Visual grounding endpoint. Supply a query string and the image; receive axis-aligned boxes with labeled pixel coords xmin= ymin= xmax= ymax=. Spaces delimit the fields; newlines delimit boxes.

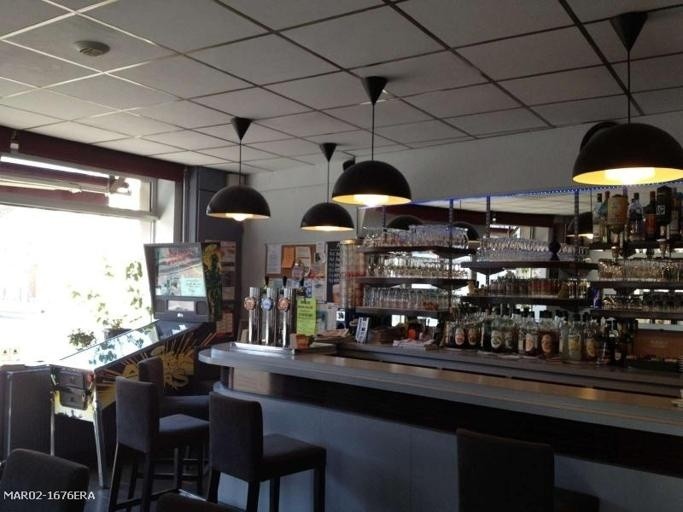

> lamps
xmin=300 ymin=143 xmax=354 ymax=231
xmin=207 ymin=117 xmax=270 ymax=221
xmin=331 ymin=76 xmax=413 ymax=206
xmin=573 ymin=11 xmax=683 ymax=186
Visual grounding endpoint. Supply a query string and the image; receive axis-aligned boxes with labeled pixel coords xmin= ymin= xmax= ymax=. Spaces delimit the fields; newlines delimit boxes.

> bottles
xmin=593 ymin=186 xmax=681 ymax=244
xmin=448 ymin=260 xmax=682 ymax=365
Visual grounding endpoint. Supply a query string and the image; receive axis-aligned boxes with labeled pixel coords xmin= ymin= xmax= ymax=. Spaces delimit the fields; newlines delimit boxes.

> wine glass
xmin=364 ymin=227 xmax=469 ymax=309
xmin=477 ymin=236 xmax=575 ymax=259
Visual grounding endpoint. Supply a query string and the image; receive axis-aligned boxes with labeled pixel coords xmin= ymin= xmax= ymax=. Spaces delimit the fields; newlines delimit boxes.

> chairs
xmin=0 ymin=448 xmax=90 ymax=511
xmin=455 ymin=429 xmax=599 ymax=512
xmin=123 ymin=357 xmax=210 ymax=511
xmin=0 ymin=365 xmax=49 ymax=454
xmin=208 ymin=391 xmax=325 ymax=511
xmin=107 ymin=378 xmax=209 ymax=511
